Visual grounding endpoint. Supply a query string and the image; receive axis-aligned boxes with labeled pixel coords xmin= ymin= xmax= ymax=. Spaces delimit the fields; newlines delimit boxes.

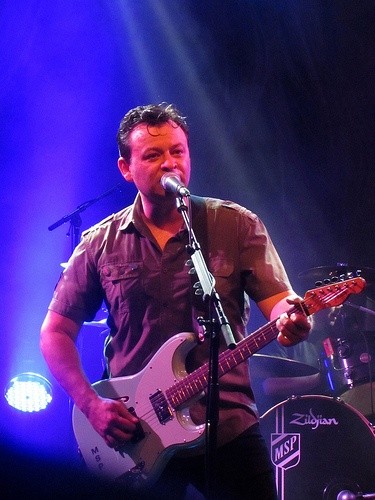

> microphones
xmin=160 ymin=172 xmax=191 ymax=197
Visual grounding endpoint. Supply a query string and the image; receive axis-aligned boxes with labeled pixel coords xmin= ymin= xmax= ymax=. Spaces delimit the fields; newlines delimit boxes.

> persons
xmin=40 ymin=102 xmax=313 ymax=500
xmin=261 ymin=304 xmax=349 ymax=398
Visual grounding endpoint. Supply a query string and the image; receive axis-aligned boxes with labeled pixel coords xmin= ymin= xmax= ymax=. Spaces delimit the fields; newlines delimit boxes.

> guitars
xmin=73 ymin=270 xmax=366 ymax=496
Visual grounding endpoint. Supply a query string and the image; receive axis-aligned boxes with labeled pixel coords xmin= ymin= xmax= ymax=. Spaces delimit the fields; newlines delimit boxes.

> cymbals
xmin=247 ymin=353 xmax=319 ymax=378
xmin=299 ymin=263 xmax=374 ymax=283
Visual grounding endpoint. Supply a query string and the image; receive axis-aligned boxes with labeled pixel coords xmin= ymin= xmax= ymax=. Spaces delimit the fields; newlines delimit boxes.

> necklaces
xmin=179 ymin=221 xmax=185 ymax=232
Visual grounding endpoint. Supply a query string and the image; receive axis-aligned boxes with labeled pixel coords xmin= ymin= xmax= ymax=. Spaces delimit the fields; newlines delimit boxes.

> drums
xmin=256 ymin=395 xmax=375 ymax=500
xmin=331 ymin=378 xmax=374 ymax=428
